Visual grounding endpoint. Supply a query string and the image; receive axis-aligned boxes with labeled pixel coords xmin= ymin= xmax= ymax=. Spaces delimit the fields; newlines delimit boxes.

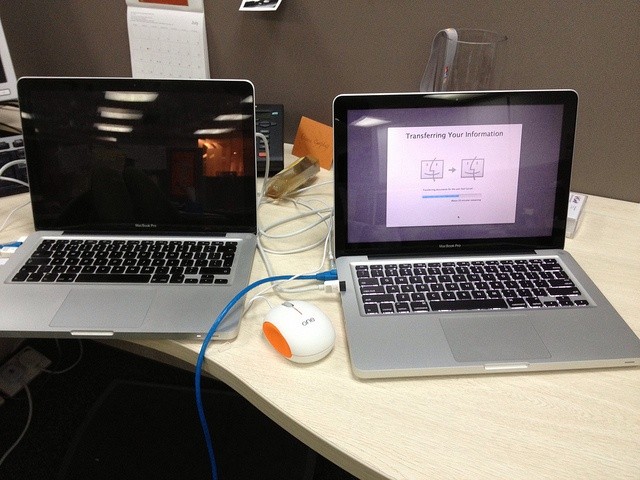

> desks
xmin=0 ymin=143 xmax=640 ymax=479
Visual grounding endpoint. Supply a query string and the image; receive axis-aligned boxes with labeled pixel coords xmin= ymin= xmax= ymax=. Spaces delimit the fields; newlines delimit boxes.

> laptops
xmin=1 ymin=75 xmax=258 ymax=341
xmin=331 ymin=88 xmax=640 ymax=380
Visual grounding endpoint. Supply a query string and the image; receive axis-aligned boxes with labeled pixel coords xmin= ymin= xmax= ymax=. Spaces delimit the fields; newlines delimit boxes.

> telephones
xmin=254 ymin=103 xmax=284 ymax=177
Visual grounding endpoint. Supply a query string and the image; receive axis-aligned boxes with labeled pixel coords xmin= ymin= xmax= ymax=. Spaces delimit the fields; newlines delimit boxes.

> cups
xmin=419 ymin=29 xmax=509 ymax=92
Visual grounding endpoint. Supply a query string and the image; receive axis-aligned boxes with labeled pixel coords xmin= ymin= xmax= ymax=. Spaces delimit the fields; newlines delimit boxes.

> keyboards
xmin=0 ymin=148 xmax=29 ymax=198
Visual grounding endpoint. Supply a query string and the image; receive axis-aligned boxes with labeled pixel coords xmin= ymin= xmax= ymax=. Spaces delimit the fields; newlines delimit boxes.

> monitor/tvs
xmin=0 ymin=18 xmax=18 ymax=102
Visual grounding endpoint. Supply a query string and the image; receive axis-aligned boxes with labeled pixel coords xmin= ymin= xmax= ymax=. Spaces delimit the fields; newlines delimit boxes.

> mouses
xmin=263 ymin=300 xmax=335 ymax=364
